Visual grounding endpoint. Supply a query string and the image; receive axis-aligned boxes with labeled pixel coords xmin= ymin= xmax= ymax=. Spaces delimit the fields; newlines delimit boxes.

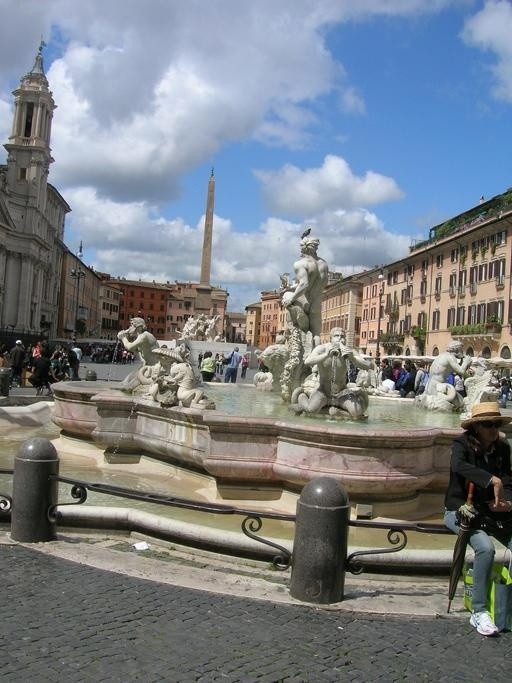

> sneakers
xmin=469 ymin=610 xmax=499 ymax=636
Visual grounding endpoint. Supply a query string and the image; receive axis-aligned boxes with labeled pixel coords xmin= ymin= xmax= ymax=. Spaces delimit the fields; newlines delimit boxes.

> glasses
xmin=478 ymin=419 xmax=503 ymax=429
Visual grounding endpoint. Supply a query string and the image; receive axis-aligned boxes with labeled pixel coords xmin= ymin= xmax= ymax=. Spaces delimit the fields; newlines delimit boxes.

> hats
xmin=461 ymin=402 xmax=511 ymax=428
xmin=16 ymin=340 xmax=22 ymax=345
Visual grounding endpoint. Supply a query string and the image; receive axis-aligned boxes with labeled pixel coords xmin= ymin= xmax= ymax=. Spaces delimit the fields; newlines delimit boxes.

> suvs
xmin=47 ymin=337 xmax=76 ymax=356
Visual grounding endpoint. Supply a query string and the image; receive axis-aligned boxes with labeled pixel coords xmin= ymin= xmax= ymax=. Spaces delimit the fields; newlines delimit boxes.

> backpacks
xmin=222 ymin=351 xmax=234 ymax=364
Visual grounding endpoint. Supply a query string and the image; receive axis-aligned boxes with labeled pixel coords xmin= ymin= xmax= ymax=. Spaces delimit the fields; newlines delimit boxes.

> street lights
xmin=266 ymin=317 xmax=271 ymax=346
xmin=375 ymin=272 xmax=385 ymax=366
xmin=70 ymin=236 xmax=87 ymax=349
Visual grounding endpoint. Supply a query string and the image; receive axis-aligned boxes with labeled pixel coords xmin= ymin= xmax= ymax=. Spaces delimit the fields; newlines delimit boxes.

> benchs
xmin=415 ymin=519 xmax=458 ymax=536
xmin=85 ymin=479 xmax=237 ymax=520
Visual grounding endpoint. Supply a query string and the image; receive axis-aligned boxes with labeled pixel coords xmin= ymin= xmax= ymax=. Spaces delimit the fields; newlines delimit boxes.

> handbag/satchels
xmin=455 ymin=499 xmax=511 ymax=538
xmin=463 ymin=548 xmax=512 ymax=633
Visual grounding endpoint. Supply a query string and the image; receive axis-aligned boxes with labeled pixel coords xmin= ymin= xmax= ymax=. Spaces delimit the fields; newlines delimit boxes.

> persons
xmin=197 ymin=347 xmax=249 ymax=383
xmin=443 ymin=402 xmax=512 ymax=637
xmin=170 ymin=343 xmax=205 ymax=409
xmin=281 ymin=237 xmax=329 ymax=334
xmin=381 ymin=357 xmax=512 ymax=402
xmin=423 ymin=340 xmax=473 ymax=407
xmin=0 ymin=339 xmax=136 ymax=395
xmin=117 ymin=317 xmax=162 ymax=387
xmin=298 ymin=327 xmax=373 ymax=417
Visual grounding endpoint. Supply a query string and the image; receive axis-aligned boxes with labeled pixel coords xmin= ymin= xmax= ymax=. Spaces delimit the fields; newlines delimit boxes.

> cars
xmin=90 ymin=350 xmax=107 ymax=361
